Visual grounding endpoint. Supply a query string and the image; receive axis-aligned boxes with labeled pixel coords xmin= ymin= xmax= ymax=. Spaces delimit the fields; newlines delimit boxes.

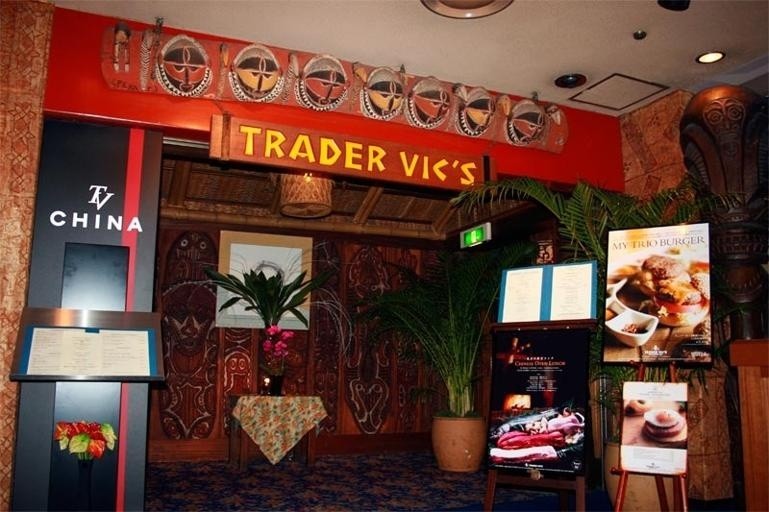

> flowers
xmin=185 ymin=240 xmax=354 ymax=366
xmin=53 ymin=420 xmax=118 ymax=460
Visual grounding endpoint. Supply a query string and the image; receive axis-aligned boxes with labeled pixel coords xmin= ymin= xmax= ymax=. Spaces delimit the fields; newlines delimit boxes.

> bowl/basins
xmin=605 ymin=308 xmax=658 ymax=348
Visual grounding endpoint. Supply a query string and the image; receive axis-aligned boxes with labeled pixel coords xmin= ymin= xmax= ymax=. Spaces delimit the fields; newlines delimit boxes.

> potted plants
xmin=448 ymin=174 xmax=747 ymax=512
xmin=354 ymin=239 xmax=540 ymax=473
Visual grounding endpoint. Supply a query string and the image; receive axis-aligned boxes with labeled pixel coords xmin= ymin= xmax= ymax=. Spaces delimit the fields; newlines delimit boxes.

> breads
xmin=603 ymin=251 xmax=710 ymax=326
xmin=624 ymin=398 xmax=688 ymax=443
xmin=489 ymin=405 xmax=588 ymax=468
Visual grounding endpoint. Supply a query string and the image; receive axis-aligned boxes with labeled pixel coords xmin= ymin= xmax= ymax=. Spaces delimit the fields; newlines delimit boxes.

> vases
xmin=259 ymin=353 xmax=289 ymax=396
xmin=77 ymin=460 xmax=93 ymax=512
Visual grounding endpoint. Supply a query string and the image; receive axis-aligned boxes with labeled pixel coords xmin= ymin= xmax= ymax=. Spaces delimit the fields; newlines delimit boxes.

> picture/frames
xmin=215 ymin=230 xmax=313 ymax=330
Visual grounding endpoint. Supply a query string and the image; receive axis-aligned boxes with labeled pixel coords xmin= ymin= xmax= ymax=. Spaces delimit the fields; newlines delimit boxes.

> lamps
xmin=279 ymin=167 xmax=335 ymax=219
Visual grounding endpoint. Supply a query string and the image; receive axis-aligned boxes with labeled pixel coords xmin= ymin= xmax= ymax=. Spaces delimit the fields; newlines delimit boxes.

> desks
xmin=228 ymin=393 xmax=328 ymax=473
